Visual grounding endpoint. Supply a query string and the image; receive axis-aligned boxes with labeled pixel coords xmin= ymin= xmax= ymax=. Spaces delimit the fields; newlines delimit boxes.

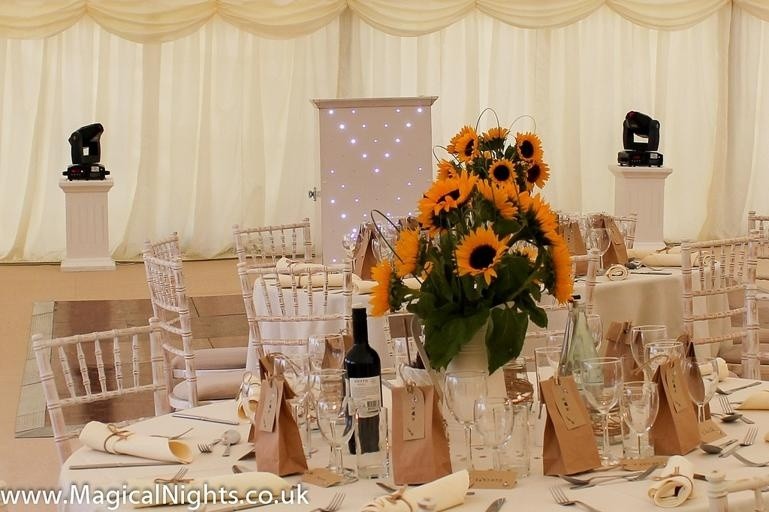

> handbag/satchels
xmin=391 ymin=387 xmax=452 ymax=485
xmin=648 ymin=357 xmax=701 ymax=456
xmin=590 ymin=214 xmax=629 ymax=268
xmin=321 ymin=329 xmax=354 ymax=415
xmin=559 ymin=221 xmax=588 ymax=277
xmin=539 ymin=375 xmax=601 ymax=476
xmin=247 ymin=353 xmax=299 ymax=443
xmin=352 ymin=224 xmax=381 ymax=281
xmin=255 ymin=378 xmax=308 ymax=476
xmin=605 ymin=321 xmax=643 ymax=387
xmin=671 ymin=335 xmax=711 ymax=423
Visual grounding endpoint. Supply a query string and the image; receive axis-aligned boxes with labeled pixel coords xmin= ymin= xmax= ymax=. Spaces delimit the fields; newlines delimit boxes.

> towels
xmin=648 ymin=455 xmax=695 ymax=508
xmin=360 ymin=470 xmax=469 ymax=512
xmin=132 ymin=471 xmax=290 ymax=507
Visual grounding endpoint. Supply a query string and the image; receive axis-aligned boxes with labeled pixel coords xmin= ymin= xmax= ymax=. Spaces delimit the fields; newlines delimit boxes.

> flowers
xmin=367 ymin=108 xmax=576 ymax=377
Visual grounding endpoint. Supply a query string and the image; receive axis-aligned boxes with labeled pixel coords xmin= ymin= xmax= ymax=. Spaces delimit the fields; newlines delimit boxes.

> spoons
xmin=231 ymin=464 xmax=253 ymax=475
xmin=699 ymin=440 xmax=737 ymax=454
xmin=221 ymin=429 xmax=241 ymax=456
xmin=376 ymin=481 xmax=476 ymax=497
xmin=711 ymin=412 xmax=743 ymax=423
xmin=556 ymin=473 xmax=644 ymax=485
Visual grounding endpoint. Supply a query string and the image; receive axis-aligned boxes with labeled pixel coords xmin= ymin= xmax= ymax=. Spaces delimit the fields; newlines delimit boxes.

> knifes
xmin=210 ymin=498 xmax=290 ymax=512
xmin=172 ymin=411 xmax=239 ymax=427
xmin=485 ymin=497 xmax=507 ymax=512
xmin=68 ymin=461 xmax=183 ymax=470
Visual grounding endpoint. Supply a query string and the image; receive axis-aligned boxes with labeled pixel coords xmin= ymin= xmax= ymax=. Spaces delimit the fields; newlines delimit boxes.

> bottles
xmin=343 ymin=307 xmax=383 ymax=455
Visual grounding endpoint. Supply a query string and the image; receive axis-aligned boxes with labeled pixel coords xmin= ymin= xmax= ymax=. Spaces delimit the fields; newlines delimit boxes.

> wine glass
xmin=580 ymin=356 xmax=624 ymax=457
xmin=586 ymin=313 xmax=603 ymax=353
xmin=544 ymin=334 xmax=563 ymax=369
xmin=473 ymin=397 xmax=515 ymax=471
xmin=618 ymin=380 xmax=660 ymax=456
xmin=443 ymin=369 xmax=489 ymax=467
xmin=341 ymin=216 xmax=399 ymax=269
xmin=557 ymin=210 xmax=612 ymax=273
xmin=629 ymin=324 xmax=667 ymax=371
xmin=308 ymin=368 xmax=351 ymax=475
xmin=642 ymin=341 xmax=684 ymax=378
xmin=683 ymin=356 xmax=719 ymax=423
xmin=315 ymin=393 xmax=359 ymax=487
xmin=307 ymin=333 xmax=346 ymax=370
xmin=273 ymin=353 xmax=315 ymax=423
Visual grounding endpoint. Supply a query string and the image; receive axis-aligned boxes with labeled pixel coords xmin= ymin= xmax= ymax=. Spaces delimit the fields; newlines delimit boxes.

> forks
xmin=170 ymin=466 xmax=189 ymax=481
xmin=717 ymin=381 xmax=762 ymax=395
xmin=568 ymin=463 xmax=659 ymax=490
xmin=718 ymin=427 xmax=759 ymax=458
xmin=149 ymin=427 xmax=194 ymax=440
xmin=310 ymin=491 xmax=346 ymax=512
xmin=549 ymin=485 xmax=601 ymax=511
xmin=718 ymin=395 xmax=754 ymax=424
xmin=197 ymin=437 xmax=221 ymax=454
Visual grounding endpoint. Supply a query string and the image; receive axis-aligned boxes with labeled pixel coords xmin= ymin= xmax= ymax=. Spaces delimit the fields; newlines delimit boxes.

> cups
xmin=533 ymin=346 xmax=561 ymax=400
xmin=355 ymin=406 xmax=389 ymax=480
xmin=492 ymin=404 xmax=531 ymax=480
